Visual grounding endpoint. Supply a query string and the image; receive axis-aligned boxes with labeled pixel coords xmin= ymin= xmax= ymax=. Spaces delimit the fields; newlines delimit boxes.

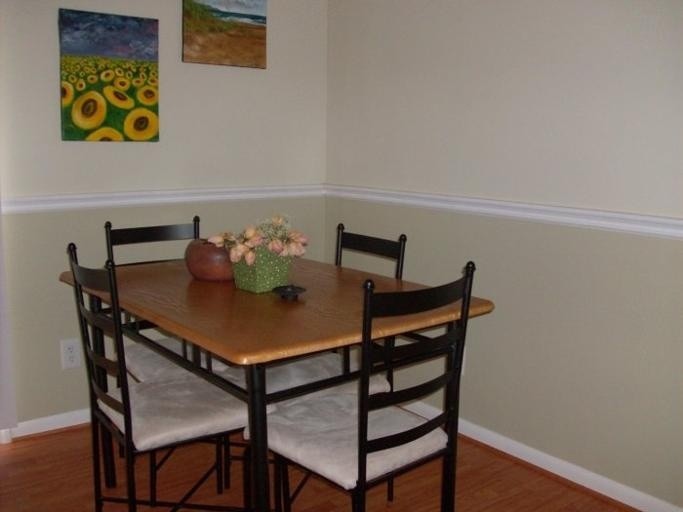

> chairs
xmin=243 ymin=257 xmax=475 ymax=512
xmin=68 ymin=242 xmax=283 ymax=512
xmin=259 ymin=222 xmax=407 ymax=501
xmin=104 ymin=218 xmax=240 ymax=490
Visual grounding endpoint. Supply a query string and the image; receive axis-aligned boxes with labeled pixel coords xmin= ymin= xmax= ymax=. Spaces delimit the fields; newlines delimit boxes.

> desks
xmin=61 ymin=253 xmax=494 ymax=512
xmin=58 ymin=257 xmax=495 ymax=512
